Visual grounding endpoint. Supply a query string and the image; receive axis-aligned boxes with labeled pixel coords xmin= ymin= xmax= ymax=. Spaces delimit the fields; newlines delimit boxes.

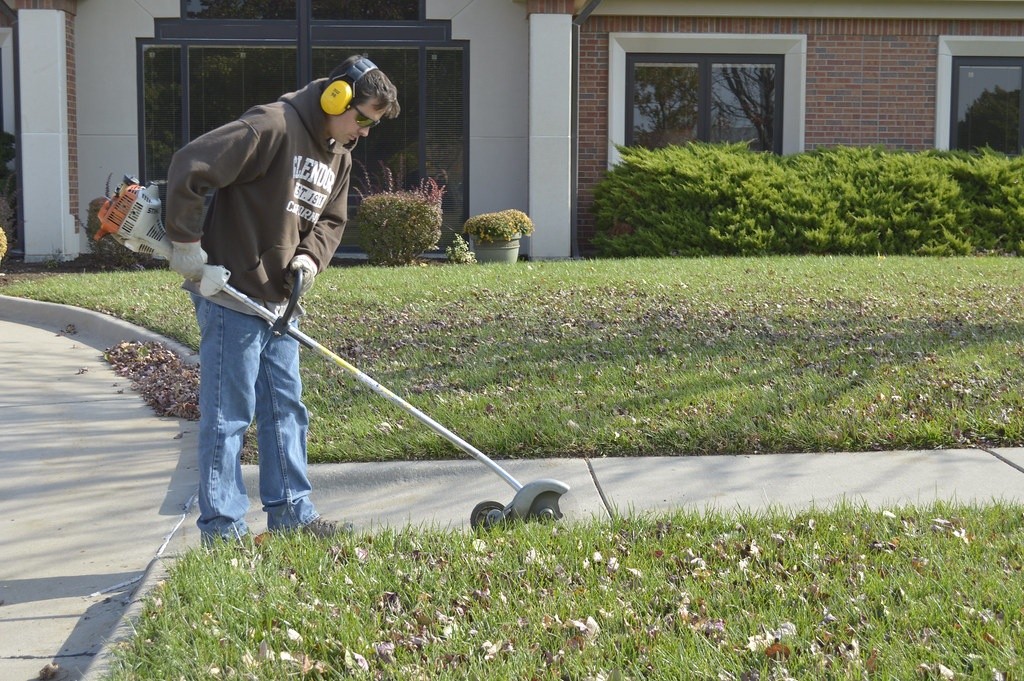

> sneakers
xmin=302 ymin=518 xmax=356 ymax=538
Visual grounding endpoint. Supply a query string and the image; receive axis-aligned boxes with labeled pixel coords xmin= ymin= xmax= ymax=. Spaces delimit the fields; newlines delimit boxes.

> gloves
xmin=169 ymin=239 xmax=208 ymax=279
xmin=283 ymin=253 xmax=319 ymax=296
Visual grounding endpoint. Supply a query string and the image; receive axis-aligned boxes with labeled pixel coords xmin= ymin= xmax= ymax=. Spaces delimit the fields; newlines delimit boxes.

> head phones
xmin=318 ymin=58 xmax=378 ymax=116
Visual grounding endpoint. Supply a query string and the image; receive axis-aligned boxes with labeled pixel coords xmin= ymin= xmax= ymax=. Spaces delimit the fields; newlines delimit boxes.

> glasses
xmin=352 ymin=104 xmax=380 ymax=129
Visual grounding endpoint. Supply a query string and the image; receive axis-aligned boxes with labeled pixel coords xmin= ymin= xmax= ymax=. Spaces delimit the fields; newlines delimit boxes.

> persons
xmin=164 ymin=55 xmax=401 ymax=551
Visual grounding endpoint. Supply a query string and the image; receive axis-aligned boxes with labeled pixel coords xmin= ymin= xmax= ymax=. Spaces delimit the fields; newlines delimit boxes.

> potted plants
xmin=461 ymin=209 xmax=535 ymax=264
